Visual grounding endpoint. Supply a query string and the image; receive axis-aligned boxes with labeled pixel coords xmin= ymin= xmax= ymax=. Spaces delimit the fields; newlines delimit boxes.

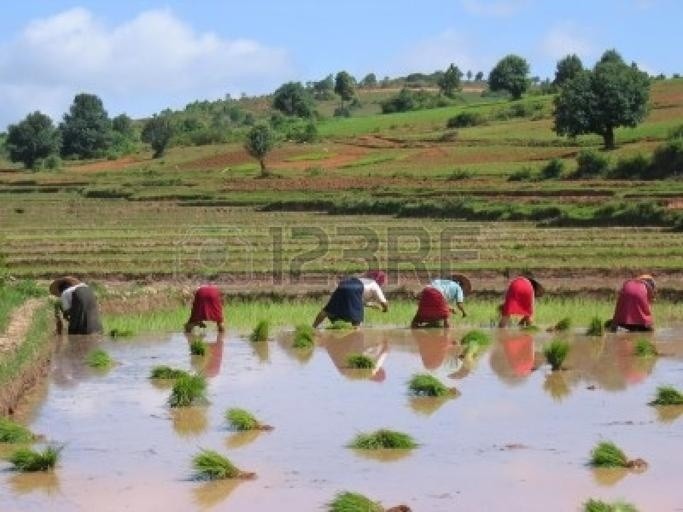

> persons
xmin=492 ymin=273 xmax=546 ymax=330
xmin=605 ymin=272 xmax=658 ymax=335
xmin=310 ymin=266 xmax=389 ymax=330
xmin=488 ymin=329 xmax=546 ymax=381
xmin=181 ymin=282 xmax=226 ymax=336
xmin=47 ymin=275 xmax=106 ymax=336
xmin=408 ymin=274 xmax=473 ymax=329
xmin=316 ymin=328 xmax=390 ymax=384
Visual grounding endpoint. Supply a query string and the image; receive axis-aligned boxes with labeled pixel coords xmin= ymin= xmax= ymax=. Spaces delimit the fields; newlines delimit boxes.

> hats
xmin=49 ymin=276 xmax=80 ymax=298
xmin=444 ymin=271 xmax=472 ymax=298
